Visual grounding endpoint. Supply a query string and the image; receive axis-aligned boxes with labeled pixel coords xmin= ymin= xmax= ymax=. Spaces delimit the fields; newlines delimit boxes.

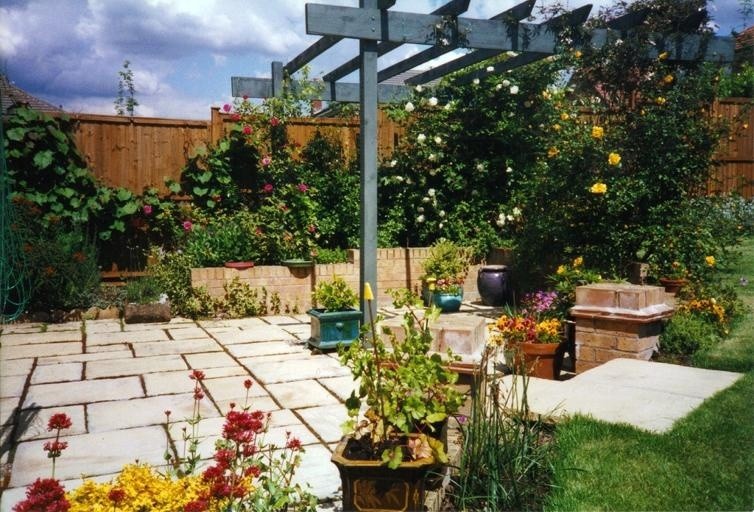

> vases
xmin=515 ymin=341 xmax=563 ymax=381
xmin=432 ymin=291 xmax=463 ymax=312
xmin=477 ymin=263 xmax=510 ymax=307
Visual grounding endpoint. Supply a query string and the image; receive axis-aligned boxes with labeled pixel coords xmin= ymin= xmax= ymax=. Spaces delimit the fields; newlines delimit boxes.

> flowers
xmin=433 ymin=276 xmax=466 ymax=297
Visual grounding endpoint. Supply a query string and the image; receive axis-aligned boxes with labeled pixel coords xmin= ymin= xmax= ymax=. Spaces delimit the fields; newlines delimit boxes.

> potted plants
xmin=330 ymin=293 xmax=473 ymax=512
xmin=306 ymin=272 xmax=365 ymax=355
